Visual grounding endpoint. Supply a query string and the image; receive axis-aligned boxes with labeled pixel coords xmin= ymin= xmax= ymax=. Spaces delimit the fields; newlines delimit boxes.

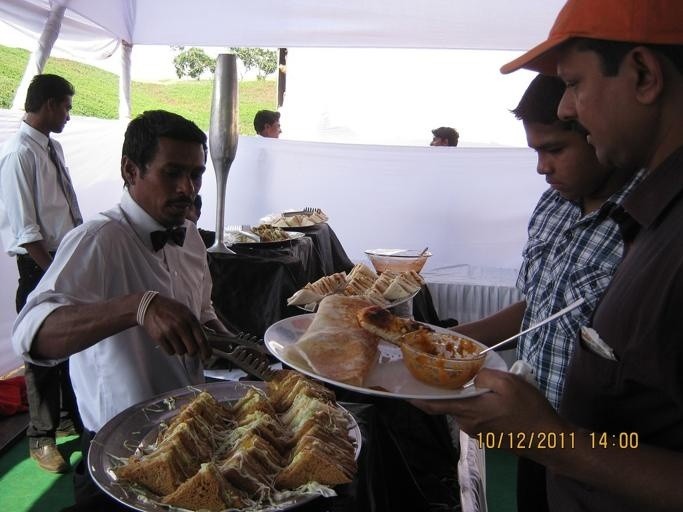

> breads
xmin=286 ymin=293 xmax=386 ymax=387
xmin=356 ymin=304 xmax=435 ymax=347
xmin=248 ymin=223 xmax=291 ymax=242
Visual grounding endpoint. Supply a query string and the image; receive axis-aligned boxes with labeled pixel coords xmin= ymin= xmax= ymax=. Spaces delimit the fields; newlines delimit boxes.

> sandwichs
xmin=261 ymin=206 xmax=328 ymax=228
xmin=286 ymin=262 xmax=427 ymax=307
xmin=113 ymin=368 xmax=359 ymax=512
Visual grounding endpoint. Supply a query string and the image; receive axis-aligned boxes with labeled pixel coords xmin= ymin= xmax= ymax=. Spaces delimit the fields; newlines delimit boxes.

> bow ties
xmin=149 ymin=227 xmax=186 ymax=253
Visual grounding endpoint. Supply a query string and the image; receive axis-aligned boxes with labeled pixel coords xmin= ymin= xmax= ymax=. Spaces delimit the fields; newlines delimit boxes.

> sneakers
xmin=28 ymin=443 xmax=68 ymax=473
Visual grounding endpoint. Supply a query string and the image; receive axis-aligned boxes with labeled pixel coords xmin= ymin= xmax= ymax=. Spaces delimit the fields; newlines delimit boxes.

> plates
xmin=225 ymin=231 xmax=306 ymax=244
xmin=87 ymin=378 xmax=363 ymax=511
xmin=295 ymin=280 xmax=419 ymax=312
xmin=264 ymin=314 xmax=509 ymax=401
xmin=261 ymin=211 xmax=329 ymax=227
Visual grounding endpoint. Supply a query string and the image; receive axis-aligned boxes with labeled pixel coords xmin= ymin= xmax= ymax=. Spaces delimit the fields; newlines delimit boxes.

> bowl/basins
xmin=402 ymin=331 xmax=489 ymax=388
xmin=364 ymin=248 xmax=432 ymax=274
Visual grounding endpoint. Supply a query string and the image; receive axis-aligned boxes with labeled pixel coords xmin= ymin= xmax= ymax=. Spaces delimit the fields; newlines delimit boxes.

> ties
xmin=47 ymin=139 xmax=83 ymax=227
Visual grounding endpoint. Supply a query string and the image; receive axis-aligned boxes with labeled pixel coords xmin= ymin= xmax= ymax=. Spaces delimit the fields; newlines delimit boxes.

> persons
xmin=429 ymin=126 xmax=458 ymax=148
xmin=0 ymin=72 xmax=93 ymax=473
xmin=185 ymin=194 xmax=215 ymax=249
xmin=402 ymin=1 xmax=681 ymax=509
xmin=252 ymin=109 xmax=283 ymax=140
xmin=430 ymin=69 xmax=651 ymax=512
xmin=9 ymin=110 xmax=233 ymax=512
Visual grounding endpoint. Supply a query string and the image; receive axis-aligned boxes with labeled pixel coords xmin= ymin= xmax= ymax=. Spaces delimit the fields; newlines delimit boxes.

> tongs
xmin=157 ymin=326 xmax=279 ymax=380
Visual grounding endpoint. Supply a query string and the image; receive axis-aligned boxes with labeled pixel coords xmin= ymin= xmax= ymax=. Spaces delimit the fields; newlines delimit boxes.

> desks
xmin=208 ymin=223 xmax=329 ymax=340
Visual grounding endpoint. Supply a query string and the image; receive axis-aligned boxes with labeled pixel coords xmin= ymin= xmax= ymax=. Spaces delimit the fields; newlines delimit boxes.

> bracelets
xmin=135 ymin=289 xmax=159 ymax=325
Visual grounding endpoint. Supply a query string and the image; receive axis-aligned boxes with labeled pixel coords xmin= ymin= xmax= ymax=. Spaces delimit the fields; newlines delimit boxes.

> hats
xmin=499 ymin=0 xmax=681 ymax=74
